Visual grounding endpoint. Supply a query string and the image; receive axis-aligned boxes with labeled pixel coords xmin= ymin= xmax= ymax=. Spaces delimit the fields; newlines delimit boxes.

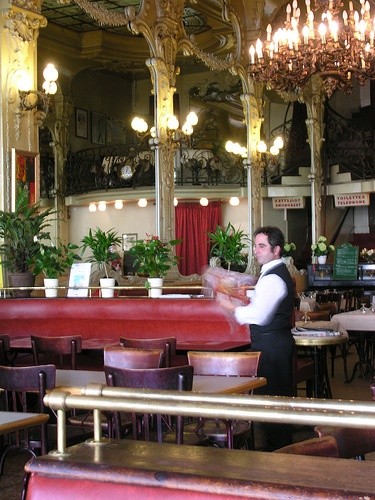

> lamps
xmin=225 ymin=137 xmax=284 ymax=168
xmin=89 ymin=197 xmax=240 ymax=212
xmin=15 ymin=63 xmax=58 ymax=121
xmin=130 ymin=112 xmax=199 ymax=147
xmin=248 ymin=0 xmax=375 ymax=97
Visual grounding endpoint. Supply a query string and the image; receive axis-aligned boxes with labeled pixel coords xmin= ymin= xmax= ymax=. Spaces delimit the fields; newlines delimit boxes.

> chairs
xmin=0 ymin=334 xmax=261 ymax=476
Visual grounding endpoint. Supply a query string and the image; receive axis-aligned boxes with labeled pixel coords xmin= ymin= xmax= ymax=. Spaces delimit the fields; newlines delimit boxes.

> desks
xmin=292 ymin=320 xmax=348 ymax=399
xmin=52 ymin=369 xmax=267 ymax=449
xmin=330 ymin=309 xmax=375 ymax=385
xmin=0 ymin=411 xmax=48 ymax=456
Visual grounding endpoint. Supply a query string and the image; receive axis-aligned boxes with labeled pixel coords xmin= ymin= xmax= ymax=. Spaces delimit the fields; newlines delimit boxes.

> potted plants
xmin=28 ymin=243 xmax=83 ymax=298
xmin=0 ymin=187 xmax=61 ymax=298
xmin=80 ymin=228 xmax=123 ymax=298
xmin=125 ymin=234 xmax=183 ymax=298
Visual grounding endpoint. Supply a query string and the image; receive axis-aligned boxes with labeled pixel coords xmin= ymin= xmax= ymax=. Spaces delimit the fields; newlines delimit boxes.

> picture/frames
xmin=91 ymin=110 xmax=106 ymax=145
xmin=76 ymin=107 xmax=88 ymax=139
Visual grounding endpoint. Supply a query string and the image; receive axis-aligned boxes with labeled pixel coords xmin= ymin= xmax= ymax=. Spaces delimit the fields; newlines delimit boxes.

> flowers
xmin=283 ymin=243 xmax=296 ymax=252
xmin=311 ymin=236 xmax=335 ymax=257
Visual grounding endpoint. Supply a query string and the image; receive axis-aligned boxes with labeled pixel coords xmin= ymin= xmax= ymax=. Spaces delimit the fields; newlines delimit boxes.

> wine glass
xmin=297 ymin=289 xmax=338 ymax=325
xmin=360 ymin=302 xmax=369 ymax=314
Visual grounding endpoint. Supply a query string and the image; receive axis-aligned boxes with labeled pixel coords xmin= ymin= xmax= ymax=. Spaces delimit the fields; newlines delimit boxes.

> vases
xmin=318 ymin=255 xmax=327 ymax=265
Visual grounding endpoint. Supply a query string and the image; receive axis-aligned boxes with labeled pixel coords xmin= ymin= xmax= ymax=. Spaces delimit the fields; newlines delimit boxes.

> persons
xmin=218 ymin=226 xmax=296 ymax=451
